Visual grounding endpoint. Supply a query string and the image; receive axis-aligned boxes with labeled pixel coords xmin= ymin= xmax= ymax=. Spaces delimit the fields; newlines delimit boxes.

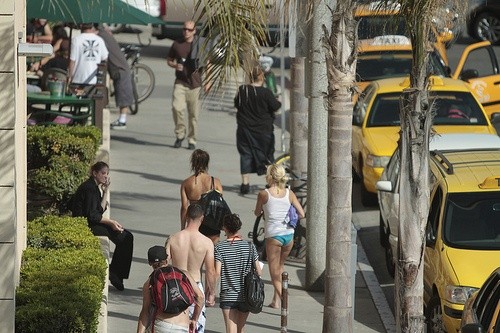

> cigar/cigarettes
xmin=121 ymin=231 xmax=123 ymax=234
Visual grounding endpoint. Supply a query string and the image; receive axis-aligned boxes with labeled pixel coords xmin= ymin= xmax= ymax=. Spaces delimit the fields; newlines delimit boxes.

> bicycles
xmin=110 ymin=45 xmax=156 ymax=104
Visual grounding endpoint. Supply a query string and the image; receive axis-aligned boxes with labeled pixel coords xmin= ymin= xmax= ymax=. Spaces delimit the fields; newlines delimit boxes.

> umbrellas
xmin=26 ymin=0 xmax=165 ymax=26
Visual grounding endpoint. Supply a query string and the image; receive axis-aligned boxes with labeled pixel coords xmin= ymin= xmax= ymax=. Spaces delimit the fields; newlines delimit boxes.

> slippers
xmin=111 ymin=119 xmax=126 ymax=126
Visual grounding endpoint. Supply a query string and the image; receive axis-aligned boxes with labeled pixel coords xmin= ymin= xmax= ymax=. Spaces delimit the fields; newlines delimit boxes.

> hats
xmin=147 ymin=245 xmax=167 ymax=264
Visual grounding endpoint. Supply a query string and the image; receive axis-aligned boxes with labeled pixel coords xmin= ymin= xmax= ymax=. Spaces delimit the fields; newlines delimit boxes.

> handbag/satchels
xmin=246 ymin=243 xmax=264 ymax=313
xmin=201 ymin=176 xmax=232 ymax=230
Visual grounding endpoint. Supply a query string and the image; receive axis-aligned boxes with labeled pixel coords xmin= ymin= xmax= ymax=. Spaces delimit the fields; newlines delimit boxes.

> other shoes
xmin=174 ymin=136 xmax=185 ymax=148
xmin=188 ymin=143 xmax=196 ymax=149
xmin=109 ymin=272 xmax=124 ymax=290
xmin=239 ymin=183 xmax=250 ymax=195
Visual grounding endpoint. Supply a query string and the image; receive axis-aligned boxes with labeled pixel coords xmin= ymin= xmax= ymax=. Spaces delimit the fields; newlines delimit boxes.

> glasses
xmin=183 ymin=28 xmax=195 ymax=32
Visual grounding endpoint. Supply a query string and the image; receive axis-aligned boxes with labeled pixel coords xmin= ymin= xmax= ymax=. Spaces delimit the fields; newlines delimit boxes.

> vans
xmin=154 ymin=1 xmax=462 ymax=68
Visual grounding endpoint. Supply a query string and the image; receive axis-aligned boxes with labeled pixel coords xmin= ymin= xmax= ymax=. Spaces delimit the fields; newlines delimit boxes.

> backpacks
xmin=150 ymin=266 xmax=198 ymax=314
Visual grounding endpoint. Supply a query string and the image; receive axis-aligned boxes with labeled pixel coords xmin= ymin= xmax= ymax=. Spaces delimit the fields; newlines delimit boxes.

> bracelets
xmin=39 ymin=61 xmax=41 ymax=66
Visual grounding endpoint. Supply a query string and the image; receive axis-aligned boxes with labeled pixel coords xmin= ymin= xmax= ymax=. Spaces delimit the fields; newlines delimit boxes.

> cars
xmin=465 ymin=0 xmax=500 ymax=46
xmin=374 ymin=132 xmax=500 ymax=333
xmin=352 ymin=1 xmax=455 ymax=73
xmin=355 ymin=39 xmax=500 ymax=123
xmin=351 ymin=77 xmax=499 ymax=208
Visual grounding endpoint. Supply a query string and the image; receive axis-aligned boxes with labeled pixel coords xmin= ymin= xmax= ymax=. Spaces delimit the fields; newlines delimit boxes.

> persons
xmin=139 ymin=246 xmax=204 ymax=333
xmin=70 ymin=162 xmax=134 ymax=291
xmin=213 ymin=215 xmax=270 ymax=333
xmin=166 ymin=205 xmax=214 ymax=333
xmin=167 ymin=20 xmax=205 ymax=150
xmin=234 ymin=67 xmax=282 ymax=195
xmin=254 ymin=164 xmax=306 ymax=309
xmin=179 ymin=150 xmax=223 ymax=305
xmin=28 ymin=17 xmax=70 ymax=77
xmin=67 ymin=22 xmax=108 ymax=97
xmin=92 ymin=24 xmax=135 ymax=129
